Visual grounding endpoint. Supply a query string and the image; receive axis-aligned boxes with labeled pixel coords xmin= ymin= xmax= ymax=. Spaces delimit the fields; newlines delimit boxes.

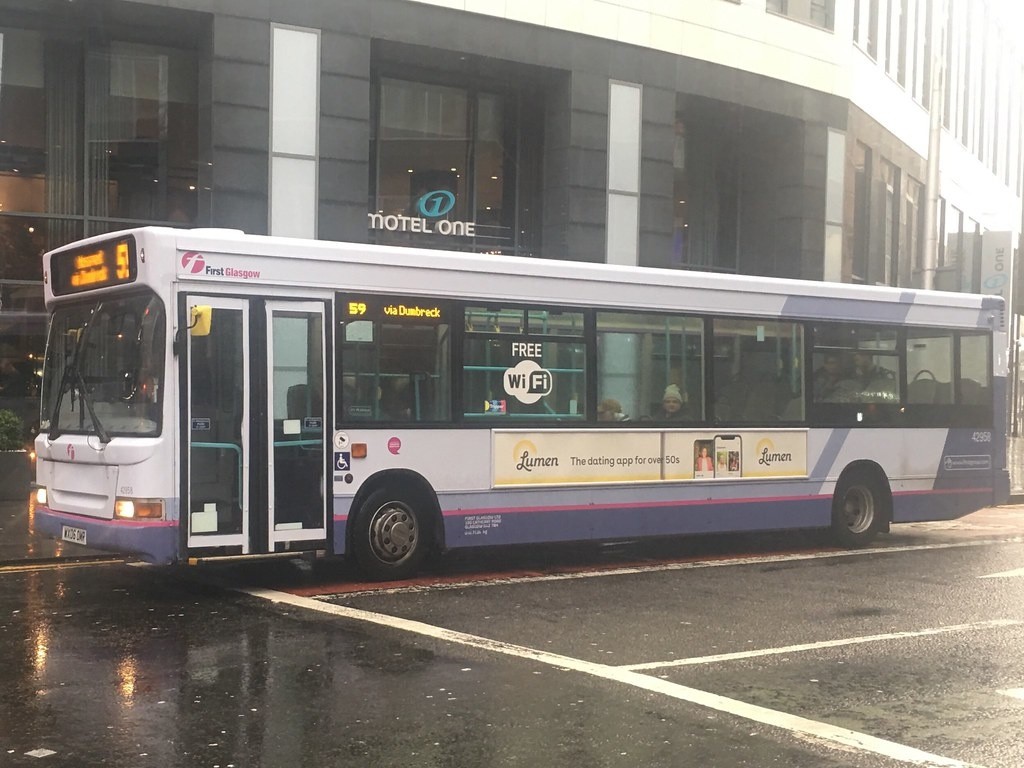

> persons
xmin=729 ymin=455 xmax=737 ymax=470
xmin=652 ymin=384 xmax=695 ymax=421
xmin=597 ymin=399 xmax=629 ymax=421
xmin=695 ymin=446 xmax=713 ymax=471
xmin=813 ymin=351 xmax=886 ymax=403
xmin=717 ymin=455 xmax=726 ymax=472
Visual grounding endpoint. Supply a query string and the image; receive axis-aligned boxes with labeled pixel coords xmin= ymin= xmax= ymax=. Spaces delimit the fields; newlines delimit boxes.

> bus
xmin=9 ymin=226 xmax=1012 ymax=583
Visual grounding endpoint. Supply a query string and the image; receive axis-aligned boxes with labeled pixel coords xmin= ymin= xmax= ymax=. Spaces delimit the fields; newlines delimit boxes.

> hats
xmin=663 ymin=384 xmax=684 ymax=405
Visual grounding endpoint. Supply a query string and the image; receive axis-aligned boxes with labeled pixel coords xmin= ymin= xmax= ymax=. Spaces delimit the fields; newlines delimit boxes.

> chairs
xmin=638 ymin=367 xmax=981 ymax=428
xmin=287 ymin=384 xmax=322 ymax=419
